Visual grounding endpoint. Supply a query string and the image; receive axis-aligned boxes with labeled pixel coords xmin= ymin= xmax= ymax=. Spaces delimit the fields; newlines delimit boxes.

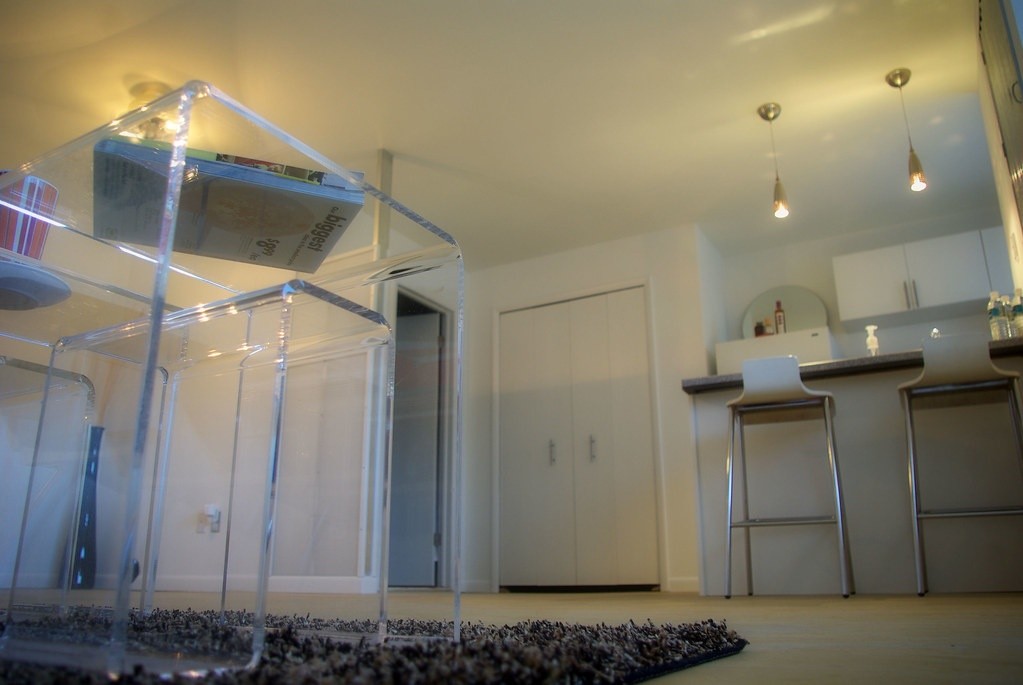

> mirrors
xmin=741 ymin=285 xmax=831 ymax=339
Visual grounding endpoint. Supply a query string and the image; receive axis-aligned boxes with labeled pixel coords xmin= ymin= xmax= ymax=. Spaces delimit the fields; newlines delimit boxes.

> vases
xmin=62 ymin=424 xmax=106 ymax=590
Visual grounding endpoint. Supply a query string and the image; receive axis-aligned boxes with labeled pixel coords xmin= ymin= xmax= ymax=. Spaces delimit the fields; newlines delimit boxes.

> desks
xmin=681 ymin=333 xmax=1023 ymax=394
xmin=0 ymin=75 xmax=468 ymax=680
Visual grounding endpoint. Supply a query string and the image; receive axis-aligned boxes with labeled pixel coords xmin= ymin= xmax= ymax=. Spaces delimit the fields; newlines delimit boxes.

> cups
xmin=0 ymin=169 xmax=60 ymax=268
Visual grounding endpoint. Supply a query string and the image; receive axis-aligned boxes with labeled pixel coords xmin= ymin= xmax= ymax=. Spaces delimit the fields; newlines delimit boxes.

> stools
xmin=896 ymin=318 xmax=1023 ymax=598
xmin=725 ymin=355 xmax=856 ymax=600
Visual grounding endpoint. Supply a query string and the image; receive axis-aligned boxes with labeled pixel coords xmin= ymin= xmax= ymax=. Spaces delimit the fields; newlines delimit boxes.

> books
xmin=93 ymin=135 xmax=365 ymax=274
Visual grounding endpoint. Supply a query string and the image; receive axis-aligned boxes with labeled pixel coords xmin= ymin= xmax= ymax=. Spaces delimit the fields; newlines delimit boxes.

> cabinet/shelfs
xmin=833 ymin=225 xmax=1014 ymax=320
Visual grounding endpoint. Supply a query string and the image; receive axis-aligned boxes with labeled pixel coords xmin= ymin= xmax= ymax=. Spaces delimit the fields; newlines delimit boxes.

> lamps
xmin=886 ymin=67 xmax=926 ymax=191
xmin=757 ymin=102 xmax=790 ymax=219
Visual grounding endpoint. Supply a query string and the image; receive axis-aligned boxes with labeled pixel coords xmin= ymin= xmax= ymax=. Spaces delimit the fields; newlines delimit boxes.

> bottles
xmin=986 ymin=287 xmax=1023 ymax=340
xmin=755 ymin=321 xmax=764 ymax=337
xmin=774 ymin=300 xmax=787 ymax=334
xmin=864 ymin=324 xmax=880 ymax=356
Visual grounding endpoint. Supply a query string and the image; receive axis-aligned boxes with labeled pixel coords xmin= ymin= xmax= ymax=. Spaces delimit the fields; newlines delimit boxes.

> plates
xmin=0 ymin=258 xmax=73 ymax=310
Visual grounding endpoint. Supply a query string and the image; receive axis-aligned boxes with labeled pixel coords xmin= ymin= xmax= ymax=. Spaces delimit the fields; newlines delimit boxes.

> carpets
xmin=0 ymin=606 xmax=749 ymax=685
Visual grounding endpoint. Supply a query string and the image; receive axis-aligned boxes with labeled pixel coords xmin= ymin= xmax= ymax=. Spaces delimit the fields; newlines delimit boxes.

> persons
xmin=238 ymin=162 xmax=282 ymax=173
